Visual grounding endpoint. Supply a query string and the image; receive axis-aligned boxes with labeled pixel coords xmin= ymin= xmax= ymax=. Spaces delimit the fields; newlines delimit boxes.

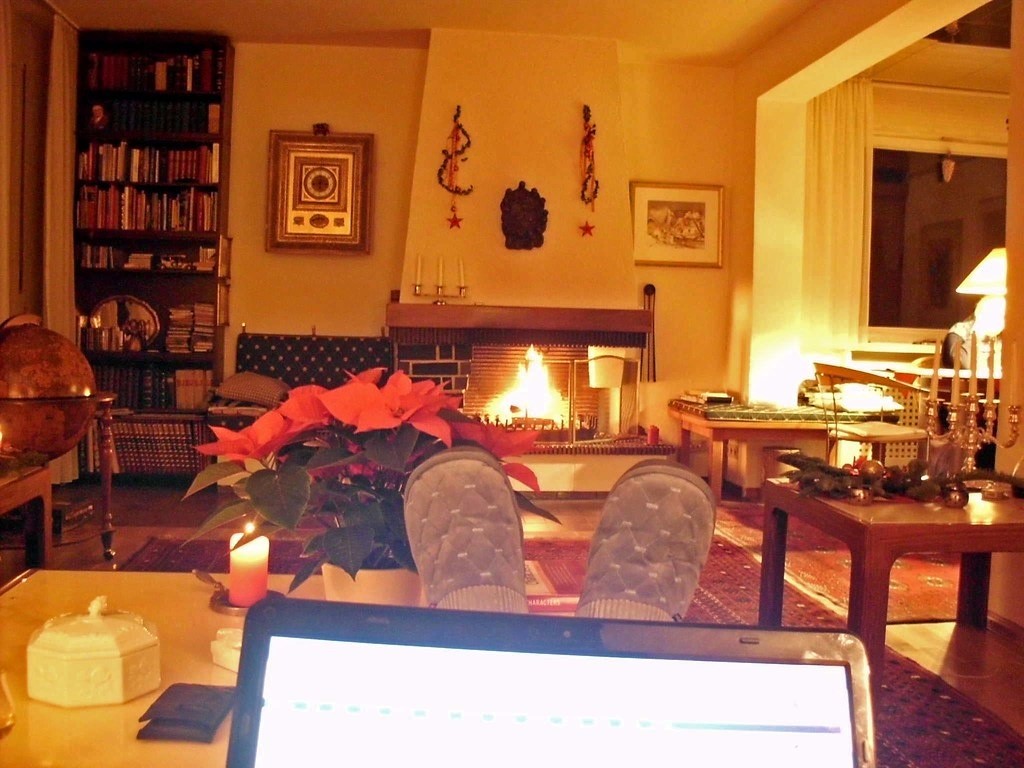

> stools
xmin=823 ymin=420 xmax=928 ymax=469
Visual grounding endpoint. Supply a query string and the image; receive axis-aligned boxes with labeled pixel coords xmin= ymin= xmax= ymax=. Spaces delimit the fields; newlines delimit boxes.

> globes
xmin=1 ymin=315 xmax=99 ymax=464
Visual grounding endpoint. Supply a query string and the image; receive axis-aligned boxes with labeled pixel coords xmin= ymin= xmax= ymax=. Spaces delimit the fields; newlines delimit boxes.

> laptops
xmin=227 ymin=598 xmax=876 ymax=768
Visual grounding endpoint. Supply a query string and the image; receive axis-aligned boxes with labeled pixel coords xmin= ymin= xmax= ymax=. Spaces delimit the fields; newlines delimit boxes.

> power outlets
xmin=728 ymin=441 xmax=739 ymax=459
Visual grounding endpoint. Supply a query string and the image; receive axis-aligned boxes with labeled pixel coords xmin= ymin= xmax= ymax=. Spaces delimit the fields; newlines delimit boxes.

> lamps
xmin=954 ymin=247 xmax=1006 ymax=373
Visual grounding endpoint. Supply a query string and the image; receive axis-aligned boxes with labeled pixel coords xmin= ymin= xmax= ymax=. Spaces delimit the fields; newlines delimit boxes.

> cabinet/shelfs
xmin=72 ymin=27 xmax=234 ymax=496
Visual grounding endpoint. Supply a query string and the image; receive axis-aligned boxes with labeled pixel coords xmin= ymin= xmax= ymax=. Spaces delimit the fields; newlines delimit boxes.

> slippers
xmin=404 ymin=446 xmax=528 ymax=615
xmin=576 ymin=457 xmax=716 ymax=623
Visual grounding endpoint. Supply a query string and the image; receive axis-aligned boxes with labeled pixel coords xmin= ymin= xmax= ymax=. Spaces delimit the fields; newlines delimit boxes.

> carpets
xmin=114 ymin=526 xmax=1024 ymax=768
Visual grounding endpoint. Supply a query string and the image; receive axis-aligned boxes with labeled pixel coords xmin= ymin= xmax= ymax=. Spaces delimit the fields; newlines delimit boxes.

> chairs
xmin=813 ymin=361 xmax=945 ymax=469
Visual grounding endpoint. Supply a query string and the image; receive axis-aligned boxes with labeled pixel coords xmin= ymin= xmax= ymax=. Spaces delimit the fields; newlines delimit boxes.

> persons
xmin=938 ymin=314 xmax=1000 ymax=470
xmin=402 ymin=448 xmax=717 ymax=622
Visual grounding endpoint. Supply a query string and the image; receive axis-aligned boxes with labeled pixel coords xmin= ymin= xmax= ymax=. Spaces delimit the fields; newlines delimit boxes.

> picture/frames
xmin=264 ymin=129 xmax=376 ymax=256
xmin=629 ymin=181 xmax=725 ymax=269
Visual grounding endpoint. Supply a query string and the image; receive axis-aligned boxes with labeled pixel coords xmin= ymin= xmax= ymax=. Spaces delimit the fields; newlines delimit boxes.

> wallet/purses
xmin=136 ymin=682 xmax=237 ymax=744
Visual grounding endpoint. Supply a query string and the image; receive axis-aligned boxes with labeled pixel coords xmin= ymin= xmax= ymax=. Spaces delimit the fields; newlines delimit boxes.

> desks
xmin=0 ymin=559 xmax=562 ymax=768
xmin=669 ymin=399 xmax=901 ymax=507
xmin=757 ymin=476 xmax=1024 ymax=722
xmin=0 ymin=464 xmax=53 ymax=570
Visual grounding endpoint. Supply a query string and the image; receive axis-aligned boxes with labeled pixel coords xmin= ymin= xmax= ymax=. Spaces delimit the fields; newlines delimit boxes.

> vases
xmin=321 ymin=564 xmax=423 ymax=606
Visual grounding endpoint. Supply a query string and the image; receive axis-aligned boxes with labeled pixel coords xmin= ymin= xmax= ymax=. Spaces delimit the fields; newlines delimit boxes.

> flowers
xmin=182 ymin=367 xmax=563 ymax=594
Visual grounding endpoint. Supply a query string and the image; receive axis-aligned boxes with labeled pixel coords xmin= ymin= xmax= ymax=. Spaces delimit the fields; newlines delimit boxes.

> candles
xmin=416 ymin=255 xmax=421 ymax=284
xmin=969 ymin=332 xmax=978 ymax=394
xmin=438 ymin=255 xmax=443 ymax=286
xmin=987 ymin=339 xmax=995 ymax=403
xmin=458 ymin=256 xmax=465 ymax=287
xmin=951 ymin=334 xmax=960 ymax=406
xmin=929 ymin=335 xmax=940 ymax=401
xmin=229 ymin=523 xmax=270 ymax=607
xmin=1008 ymin=340 xmax=1018 ymax=406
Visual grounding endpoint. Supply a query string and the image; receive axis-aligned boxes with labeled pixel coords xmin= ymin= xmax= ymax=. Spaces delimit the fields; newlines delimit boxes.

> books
xmin=78 ymin=139 xmax=219 ymax=183
xmin=86 ymin=100 xmax=220 ymax=134
xmin=75 ymin=184 xmax=218 ymax=230
xmin=87 ymin=49 xmax=223 ymax=92
xmin=683 ymin=391 xmax=733 ymax=404
xmin=75 ymin=302 xmax=216 ymax=352
xmin=78 ymin=422 xmax=212 ymax=474
xmin=81 ymin=241 xmax=217 ymax=271
